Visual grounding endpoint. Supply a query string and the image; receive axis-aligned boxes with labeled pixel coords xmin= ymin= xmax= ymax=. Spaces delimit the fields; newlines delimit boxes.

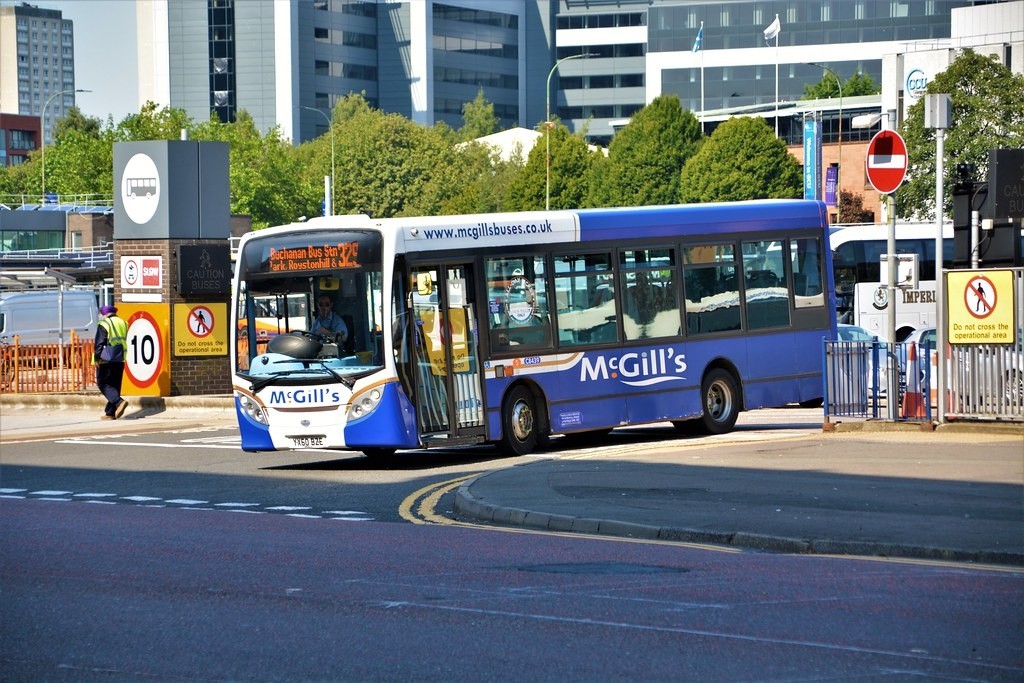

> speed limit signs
xmin=123 ymin=311 xmax=164 ymax=389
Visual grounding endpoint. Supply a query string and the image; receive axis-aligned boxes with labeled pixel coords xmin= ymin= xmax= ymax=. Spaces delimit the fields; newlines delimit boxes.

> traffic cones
xmin=901 ymin=341 xmax=963 ymax=421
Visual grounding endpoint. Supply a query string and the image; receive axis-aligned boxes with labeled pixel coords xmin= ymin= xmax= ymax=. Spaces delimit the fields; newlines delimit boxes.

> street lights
xmin=291 ymin=105 xmax=338 ymax=215
xmin=808 ymin=60 xmax=843 ymax=223
xmin=41 ymin=89 xmax=93 ymax=201
xmin=542 ymin=52 xmax=599 ymax=211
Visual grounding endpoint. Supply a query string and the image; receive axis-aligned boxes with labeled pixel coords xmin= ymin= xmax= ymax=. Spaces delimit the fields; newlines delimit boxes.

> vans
xmin=0 ymin=288 xmax=98 ymax=368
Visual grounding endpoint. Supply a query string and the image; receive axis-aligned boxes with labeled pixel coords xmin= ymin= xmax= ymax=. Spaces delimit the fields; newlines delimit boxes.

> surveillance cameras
xmin=44 ymin=267 xmax=76 ymax=284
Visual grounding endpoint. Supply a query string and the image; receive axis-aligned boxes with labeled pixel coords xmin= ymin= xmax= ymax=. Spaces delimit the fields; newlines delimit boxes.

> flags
xmin=763 ymin=16 xmax=781 ymax=40
xmin=692 ymin=27 xmax=702 ymax=53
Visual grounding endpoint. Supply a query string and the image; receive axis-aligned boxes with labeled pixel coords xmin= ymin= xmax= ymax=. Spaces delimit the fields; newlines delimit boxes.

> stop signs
xmin=864 ymin=129 xmax=908 ymax=194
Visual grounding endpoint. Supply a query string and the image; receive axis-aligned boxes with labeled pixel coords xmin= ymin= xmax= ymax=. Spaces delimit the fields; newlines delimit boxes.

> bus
xmin=829 ymin=221 xmax=955 ymax=323
xmin=227 ymin=199 xmax=837 ymax=462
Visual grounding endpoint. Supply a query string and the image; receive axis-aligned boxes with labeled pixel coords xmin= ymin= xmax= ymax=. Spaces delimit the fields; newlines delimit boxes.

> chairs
xmin=589 ymin=271 xmax=810 ymax=345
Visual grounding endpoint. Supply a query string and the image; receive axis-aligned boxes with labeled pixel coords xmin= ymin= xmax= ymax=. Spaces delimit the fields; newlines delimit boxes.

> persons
xmin=309 ymin=294 xmax=348 ymax=342
xmin=91 ymin=306 xmax=129 ymax=419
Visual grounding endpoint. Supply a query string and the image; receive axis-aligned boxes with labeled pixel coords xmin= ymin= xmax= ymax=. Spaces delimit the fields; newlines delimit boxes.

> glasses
xmin=317 ymin=302 xmax=332 ymax=308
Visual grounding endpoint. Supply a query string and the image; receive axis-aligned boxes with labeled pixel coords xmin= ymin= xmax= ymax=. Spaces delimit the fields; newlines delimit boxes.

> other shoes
xmin=114 ymin=400 xmax=129 ymax=418
xmin=101 ymin=414 xmax=115 ymax=421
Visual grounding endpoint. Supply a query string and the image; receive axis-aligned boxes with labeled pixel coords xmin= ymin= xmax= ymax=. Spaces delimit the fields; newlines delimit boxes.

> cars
xmin=894 ymin=327 xmax=1024 ymax=409
xmin=836 ymin=324 xmax=927 ymax=403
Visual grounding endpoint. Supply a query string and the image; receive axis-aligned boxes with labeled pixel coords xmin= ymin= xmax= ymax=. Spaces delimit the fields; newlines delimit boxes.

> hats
xmin=101 ymin=305 xmax=118 ymax=316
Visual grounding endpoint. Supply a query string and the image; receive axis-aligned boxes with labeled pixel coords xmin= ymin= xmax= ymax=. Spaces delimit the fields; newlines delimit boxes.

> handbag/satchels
xmin=100 ymin=343 xmax=124 ymax=362
